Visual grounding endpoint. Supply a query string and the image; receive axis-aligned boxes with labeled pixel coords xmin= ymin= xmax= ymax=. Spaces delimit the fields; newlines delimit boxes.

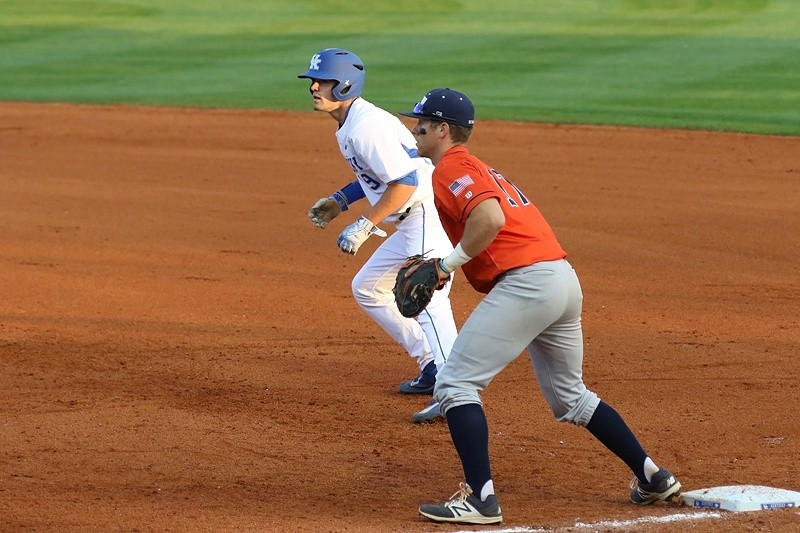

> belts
xmin=399 ymin=207 xmax=411 ymax=221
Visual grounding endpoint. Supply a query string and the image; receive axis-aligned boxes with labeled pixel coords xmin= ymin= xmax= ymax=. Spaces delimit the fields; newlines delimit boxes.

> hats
xmin=399 ymin=87 xmax=475 ymax=127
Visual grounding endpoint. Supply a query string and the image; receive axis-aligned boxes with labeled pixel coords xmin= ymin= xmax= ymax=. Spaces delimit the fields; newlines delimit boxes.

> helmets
xmin=298 ymin=48 xmax=366 ymax=101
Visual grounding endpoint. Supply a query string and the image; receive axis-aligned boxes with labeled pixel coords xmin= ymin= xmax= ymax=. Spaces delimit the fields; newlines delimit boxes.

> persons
xmin=297 ymin=48 xmax=458 ymax=424
xmin=392 ymin=88 xmax=682 ymax=525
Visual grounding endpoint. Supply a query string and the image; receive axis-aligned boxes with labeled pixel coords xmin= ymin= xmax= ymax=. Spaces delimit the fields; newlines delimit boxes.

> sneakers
xmin=419 ymin=479 xmax=502 ymax=524
xmin=630 ymin=456 xmax=681 ymax=506
xmin=398 ymin=361 xmax=438 ymax=393
xmin=412 ymin=399 xmax=443 ymax=423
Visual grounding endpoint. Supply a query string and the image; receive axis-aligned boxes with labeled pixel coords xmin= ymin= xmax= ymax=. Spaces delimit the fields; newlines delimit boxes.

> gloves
xmin=308 ymin=191 xmax=348 ymax=229
xmin=336 ymin=215 xmax=388 ymax=256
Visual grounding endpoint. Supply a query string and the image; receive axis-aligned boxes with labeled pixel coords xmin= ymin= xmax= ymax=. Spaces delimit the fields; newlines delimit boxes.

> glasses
xmin=413 ymin=101 xmax=457 ymax=122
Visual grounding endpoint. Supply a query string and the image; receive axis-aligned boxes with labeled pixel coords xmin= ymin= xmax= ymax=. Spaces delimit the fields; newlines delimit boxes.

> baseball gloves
xmin=390 ymin=247 xmax=452 ymax=319
